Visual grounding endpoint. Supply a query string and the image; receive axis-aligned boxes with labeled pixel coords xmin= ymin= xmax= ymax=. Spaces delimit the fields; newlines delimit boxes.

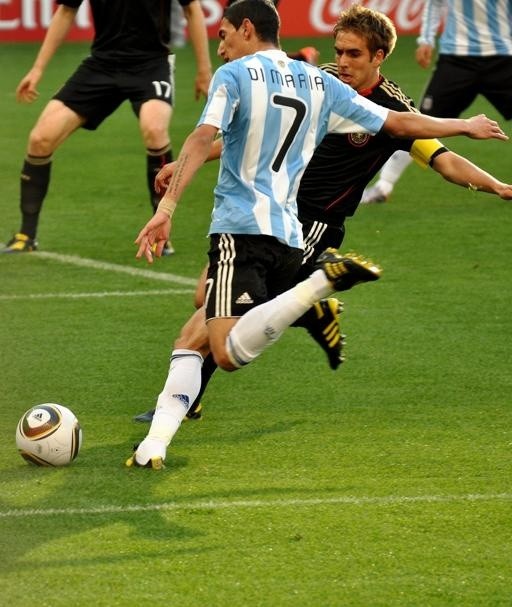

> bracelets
xmin=155 ymin=195 xmax=181 ymax=222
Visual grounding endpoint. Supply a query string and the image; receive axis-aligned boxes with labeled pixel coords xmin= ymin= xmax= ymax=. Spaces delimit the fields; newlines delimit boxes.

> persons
xmin=355 ymin=0 xmax=510 ymax=206
xmin=0 ymin=1 xmax=214 ymax=257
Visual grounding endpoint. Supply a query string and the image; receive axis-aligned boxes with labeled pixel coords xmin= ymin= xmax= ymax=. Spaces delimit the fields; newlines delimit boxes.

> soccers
xmin=15 ymin=403 xmax=82 ymax=467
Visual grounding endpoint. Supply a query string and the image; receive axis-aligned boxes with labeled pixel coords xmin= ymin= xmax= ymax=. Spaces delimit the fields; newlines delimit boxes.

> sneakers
xmin=314 ymin=247 xmax=384 ymax=292
xmin=305 ymin=297 xmax=346 ymax=371
xmin=149 ymin=239 xmax=175 ymax=255
xmin=0 ymin=232 xmax=38 ymax=253
xmin=359 ymin=181 xmax=388 ymax=204
xmin=131 ymin=403 xmax=203 ymax=423
xmin=123 ymin=453 xmax=165 ymax=471
